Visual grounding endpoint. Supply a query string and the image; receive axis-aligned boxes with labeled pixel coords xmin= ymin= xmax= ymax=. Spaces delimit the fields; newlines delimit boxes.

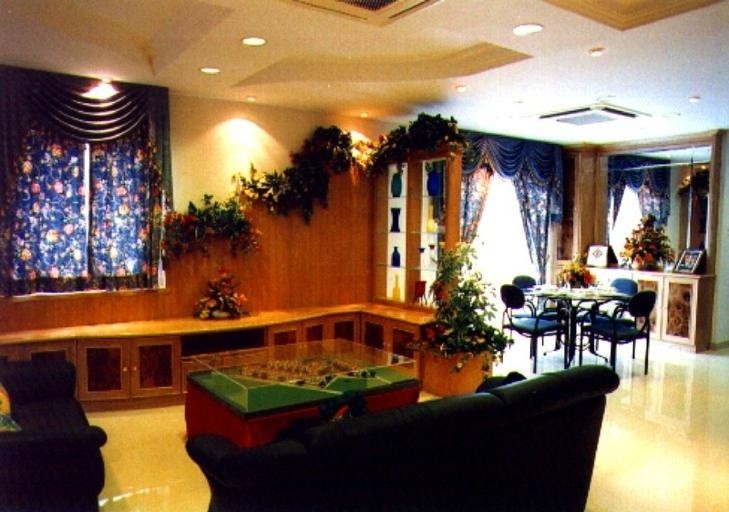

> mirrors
xmin=592 ymin=136 xmax=721 ymax=271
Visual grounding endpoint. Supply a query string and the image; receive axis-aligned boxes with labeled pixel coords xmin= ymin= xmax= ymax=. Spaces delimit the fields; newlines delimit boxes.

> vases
xmin=421 ymin=346 xmax=494 ymax=399
xmin=631 ymin=260 xmax=658 ymax=271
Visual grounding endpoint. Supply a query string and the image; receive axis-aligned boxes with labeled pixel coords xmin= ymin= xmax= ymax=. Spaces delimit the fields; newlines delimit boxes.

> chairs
xmin=0 ymin=361 xmax=107 ymax=511
xmin=500 ymin=274 xmax=656 ymax=376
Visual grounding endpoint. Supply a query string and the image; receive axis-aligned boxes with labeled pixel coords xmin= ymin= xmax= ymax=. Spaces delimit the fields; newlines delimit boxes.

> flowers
xmin=160 ymin=192 xmax=263 ymax=263
xmin=553 ymin=252 xmax=597 ymax=290
xmin=404 ymin=235 xmax=515 ymax=380
xmin=231 ymin=110 xmax=470 ymax=227
xmin=618 ymin=214 xmax=676 ymax=272
xmin=191 ymin=265 xmax=252 ymax=320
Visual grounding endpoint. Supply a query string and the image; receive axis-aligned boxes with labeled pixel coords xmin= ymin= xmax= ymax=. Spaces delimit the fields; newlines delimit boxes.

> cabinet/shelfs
xmin=585 ymin=267 xmax=716 ymax=353
xmin=1 ymin=302 xmax=494 ymax=411
xmin=373 ymin=144 xmax=462 ymax=312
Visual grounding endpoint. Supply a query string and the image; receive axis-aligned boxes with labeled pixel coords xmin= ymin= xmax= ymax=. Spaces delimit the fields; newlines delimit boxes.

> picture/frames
xmin=586 ymin=245 xmax=608 ymax=267
xmin=672 ymin=249 xmax=705 ymax=274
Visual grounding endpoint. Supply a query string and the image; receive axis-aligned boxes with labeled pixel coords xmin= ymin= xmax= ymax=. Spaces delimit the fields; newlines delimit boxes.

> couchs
xmin=185 ymin=363 xmax=620 ymax=512
